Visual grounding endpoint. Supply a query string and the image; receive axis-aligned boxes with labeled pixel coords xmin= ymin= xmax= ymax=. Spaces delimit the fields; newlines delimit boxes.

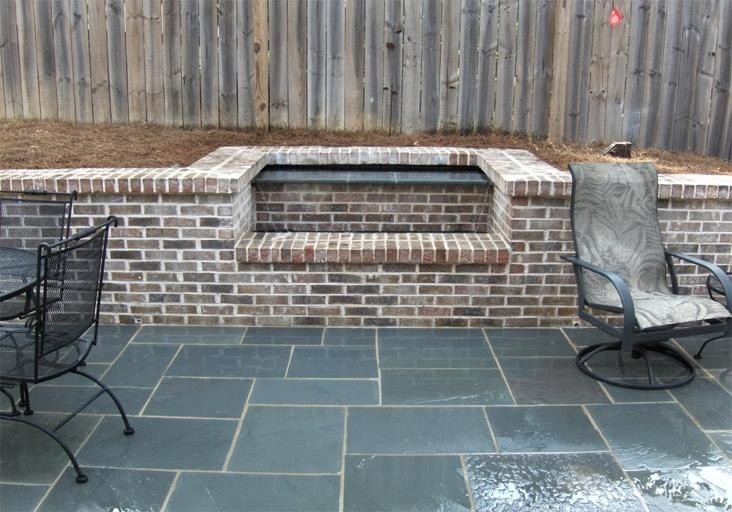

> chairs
xmin=1 ymin=188 xmax=135 ymax=485
xmin=559 ymin=159 xmax=732 ymax=390
